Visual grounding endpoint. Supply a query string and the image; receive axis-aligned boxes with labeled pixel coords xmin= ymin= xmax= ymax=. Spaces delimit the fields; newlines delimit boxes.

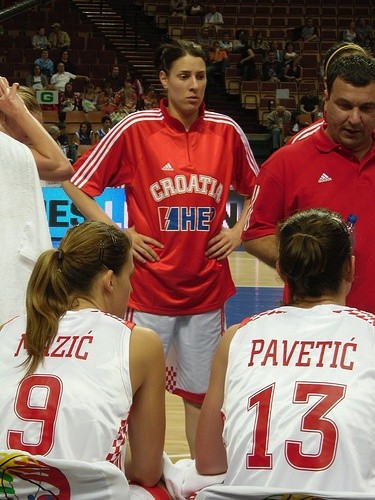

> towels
xmin=162 ymin=454 xmax=226 ymax=500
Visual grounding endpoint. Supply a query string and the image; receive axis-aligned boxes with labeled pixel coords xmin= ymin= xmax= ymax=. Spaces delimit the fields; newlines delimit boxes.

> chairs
xmin=133 ymin=0 xmax=375 ymax=128
xmin=0 ymin=0 xmax=130 ymax=158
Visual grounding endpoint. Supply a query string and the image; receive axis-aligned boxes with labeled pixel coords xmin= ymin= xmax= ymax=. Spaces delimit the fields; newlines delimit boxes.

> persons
xmin=241 ymin=53 xmax=375 ymax=315
xmin=186 ymin=209 xmax=375 ymax=500
xmin=267 ymin=86 xmax=320 ymax=147
xmin=0 ymin=75 xmax=74 ymax=320
xmin=25 ymin=22 xmax=158 ymax=163
xmin=170 ymin=0 xmax=224 ymax=32
xmin=0 ymin=219 xmax=175 ymax=500
xmin=65 ymin=39 xmax=259 ymax=463
xmin=288 ymin=41 xmax=367 ymax=146
xmin=296 ymin=18 xmax=320 ymax=41
xmin=207 ymin=29 xmax=304 ymax=85
xmin=343 ymin=17 xmax=375 ymax=53
